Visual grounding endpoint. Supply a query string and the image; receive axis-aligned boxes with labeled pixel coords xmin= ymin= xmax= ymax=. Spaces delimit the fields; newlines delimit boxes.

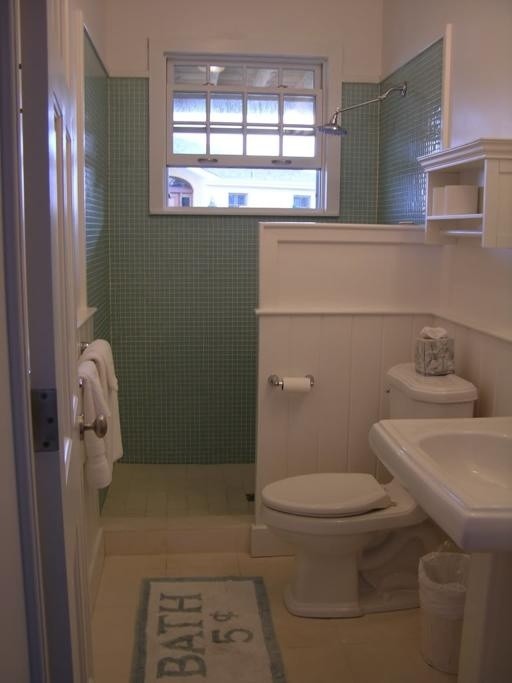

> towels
xmin=80 ymin=361 xmax=113 ymax=492
xmin=82 ymin=338 xmax=124 ymax=463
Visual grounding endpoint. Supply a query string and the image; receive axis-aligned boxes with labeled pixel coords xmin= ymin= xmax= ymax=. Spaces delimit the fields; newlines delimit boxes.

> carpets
xmin=130 ymin=577 xmax=287 ymax=683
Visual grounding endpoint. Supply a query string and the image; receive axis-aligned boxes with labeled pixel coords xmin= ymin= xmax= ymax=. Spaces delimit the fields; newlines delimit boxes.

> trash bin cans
xmin=416 ymin=548 xmax=472 ymax=674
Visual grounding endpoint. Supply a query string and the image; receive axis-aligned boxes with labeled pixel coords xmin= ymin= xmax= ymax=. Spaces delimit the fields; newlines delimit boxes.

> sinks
xmin=364 ymin=417 xmax=512 ymax=555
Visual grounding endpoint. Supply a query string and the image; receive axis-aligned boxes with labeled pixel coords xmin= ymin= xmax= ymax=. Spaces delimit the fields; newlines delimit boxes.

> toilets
xmin=257 ymin=364 xmax=480 ymax=619
